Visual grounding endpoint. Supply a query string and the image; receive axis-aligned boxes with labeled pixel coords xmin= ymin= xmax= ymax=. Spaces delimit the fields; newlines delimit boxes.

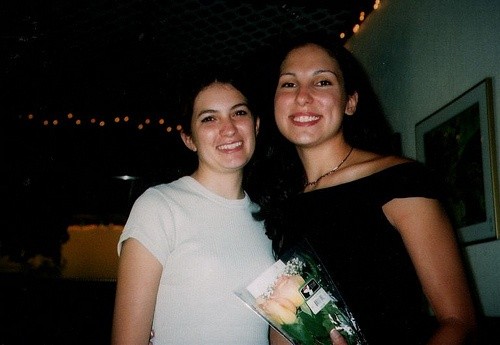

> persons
xmin=111 ymin=70 xmax=296 ymax=344
xmin=148 ymin=34 xmax=478 ymax=344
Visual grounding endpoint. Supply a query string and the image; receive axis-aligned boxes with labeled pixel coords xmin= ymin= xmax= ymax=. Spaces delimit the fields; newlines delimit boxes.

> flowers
xmin=233 ymin=246 xmax=370 ymax=345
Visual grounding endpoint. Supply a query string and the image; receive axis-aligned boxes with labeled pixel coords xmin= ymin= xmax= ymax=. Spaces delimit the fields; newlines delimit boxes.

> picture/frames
xmin=413 ymin=75 xmax=500 ymax=247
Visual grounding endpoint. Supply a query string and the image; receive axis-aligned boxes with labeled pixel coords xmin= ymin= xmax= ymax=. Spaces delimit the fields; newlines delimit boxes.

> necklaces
xmin=303 ymin=146 xmax=354 ymax=186
